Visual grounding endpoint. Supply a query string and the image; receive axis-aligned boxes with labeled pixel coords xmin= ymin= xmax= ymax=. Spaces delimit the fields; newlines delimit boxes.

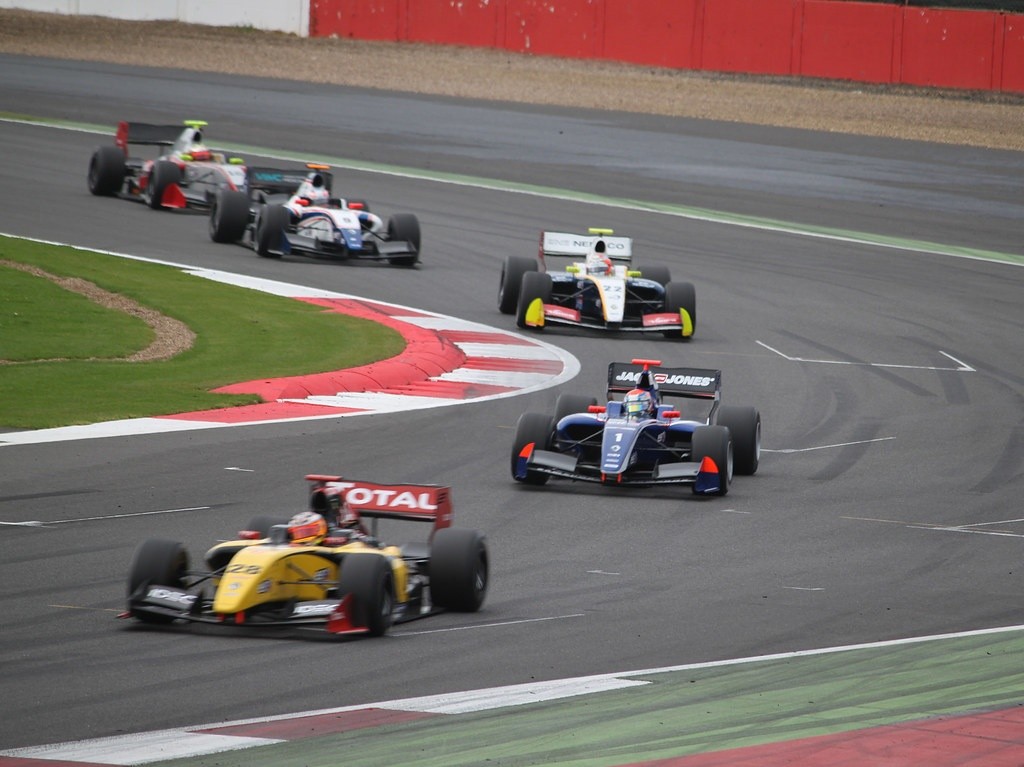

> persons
xmin=183 ymin=144 xmax=211 ymax=163
xmin=305 ymin=187 xmax=328 ymax=207
xmin=585 ymin=254 xmax=612 ymax=276
xmin=289 ymin=511 xmax=328 ymax=548
xmin=622 ymin=389 xmax=652 ymax=419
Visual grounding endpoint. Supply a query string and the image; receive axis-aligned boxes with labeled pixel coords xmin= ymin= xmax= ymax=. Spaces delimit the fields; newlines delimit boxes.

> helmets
xmin=586 ymin=253 xmax=613 ymax=277
xmin=622 ymin=389 xmax=653 ymax=417
xmin=300 ymin=186 xmax=330 ymax=207
xmin=288 ymin=512 xmax=327 ymax=546
xmin=188 ymin=144 xmax=213 ymax=161
